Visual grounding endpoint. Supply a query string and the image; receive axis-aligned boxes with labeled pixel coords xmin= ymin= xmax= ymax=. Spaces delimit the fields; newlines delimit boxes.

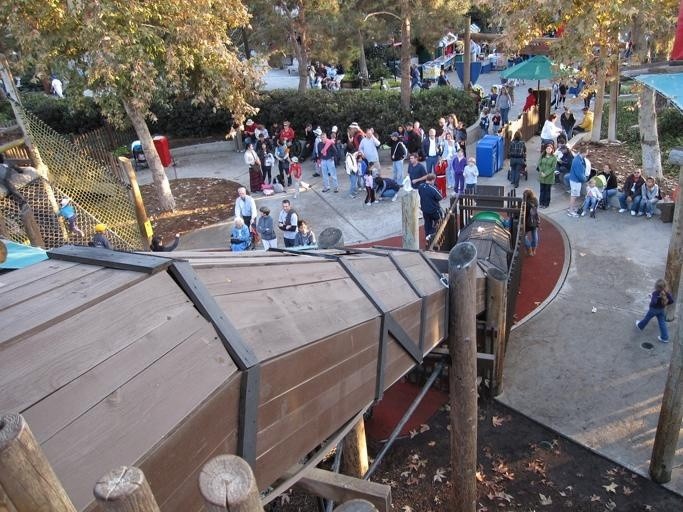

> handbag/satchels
xmin=259 ymin=174 xmax=285 ymax=197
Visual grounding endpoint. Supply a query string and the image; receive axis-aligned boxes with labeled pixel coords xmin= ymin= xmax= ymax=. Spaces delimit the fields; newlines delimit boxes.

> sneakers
xmin=657 ymin=335 xmax=669 ymax=343
xmin=313 ymin=173 xmax=320 ymax=177
xmin=638 ymin=211 xmax=645 ymax=216
xmin=630 ymin=209 xmax=636 ymax=216
xmin=321 ymin=187 xmax=385 ymax=207
xmin=619 ymin=207 xmax=628 ymax=213
xmin=635 ymin=319 xmax=642 ymax=331
xmin=646 ymin=212 xmax=652 ymax=219
xmin=567 ymin=205 xmax=607 ymax=220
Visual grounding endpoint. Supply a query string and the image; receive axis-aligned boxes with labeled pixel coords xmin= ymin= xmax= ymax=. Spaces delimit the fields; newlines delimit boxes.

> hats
xmin=389 ymin=131 xmax=400 ymax=137
xmin=290 ymin=156 xmax=299 ymax=163
xmin=246 ymin=118 xmax=254 ymax=126
xmin=348 ymin=121 xmax=360 ymax=129
xmin=331 ymin=126 xmax=338 ymax=132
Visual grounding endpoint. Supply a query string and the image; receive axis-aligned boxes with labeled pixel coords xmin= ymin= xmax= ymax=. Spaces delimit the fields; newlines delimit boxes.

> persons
xmin=49 ymin=74 xmax=65 ymax=98
xmin=409 ymin=64 xmax=450 ymax=90
xmin=149 ymin=232 xmax=180 ymax=252
xmin=90 ymin=223 xmax=110 ymax=250
xmin=53 ymin=198 xmax=84 ymax=237
xmin=307 ymin=61 xmax=345 ymax=92
xmin=470 ymin=38 xmax=481 ymax=63
xmin=635 ymin=278 xmax=673 ymax=344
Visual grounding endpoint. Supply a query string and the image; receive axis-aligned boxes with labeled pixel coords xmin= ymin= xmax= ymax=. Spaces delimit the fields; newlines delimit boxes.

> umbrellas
xmin=497 ymin=52 xmax=580 ymax=106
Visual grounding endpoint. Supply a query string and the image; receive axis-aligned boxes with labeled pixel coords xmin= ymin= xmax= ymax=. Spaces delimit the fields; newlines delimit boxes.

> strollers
xmin=576 ymin=172 xmax=610 ymax=221
xmin=508 ymin=157 xmax=529 ymax=180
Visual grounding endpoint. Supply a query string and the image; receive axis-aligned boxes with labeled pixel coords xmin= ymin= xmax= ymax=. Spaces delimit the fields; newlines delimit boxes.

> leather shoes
xmin=540 ymin=204 xmax=547 ymax=208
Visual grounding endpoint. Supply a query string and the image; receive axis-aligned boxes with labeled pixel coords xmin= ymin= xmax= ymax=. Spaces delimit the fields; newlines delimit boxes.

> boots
xmin=526 ymin=247 xmax=537 ymax=256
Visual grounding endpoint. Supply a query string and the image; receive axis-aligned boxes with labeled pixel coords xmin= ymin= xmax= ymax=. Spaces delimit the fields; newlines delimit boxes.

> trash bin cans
xmin=475 ymin=134 xmax=504 ymax=177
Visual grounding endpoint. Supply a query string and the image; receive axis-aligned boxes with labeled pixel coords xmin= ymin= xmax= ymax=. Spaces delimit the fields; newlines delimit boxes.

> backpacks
xmin=527 ymin=202 xmax=540 ymax=230
